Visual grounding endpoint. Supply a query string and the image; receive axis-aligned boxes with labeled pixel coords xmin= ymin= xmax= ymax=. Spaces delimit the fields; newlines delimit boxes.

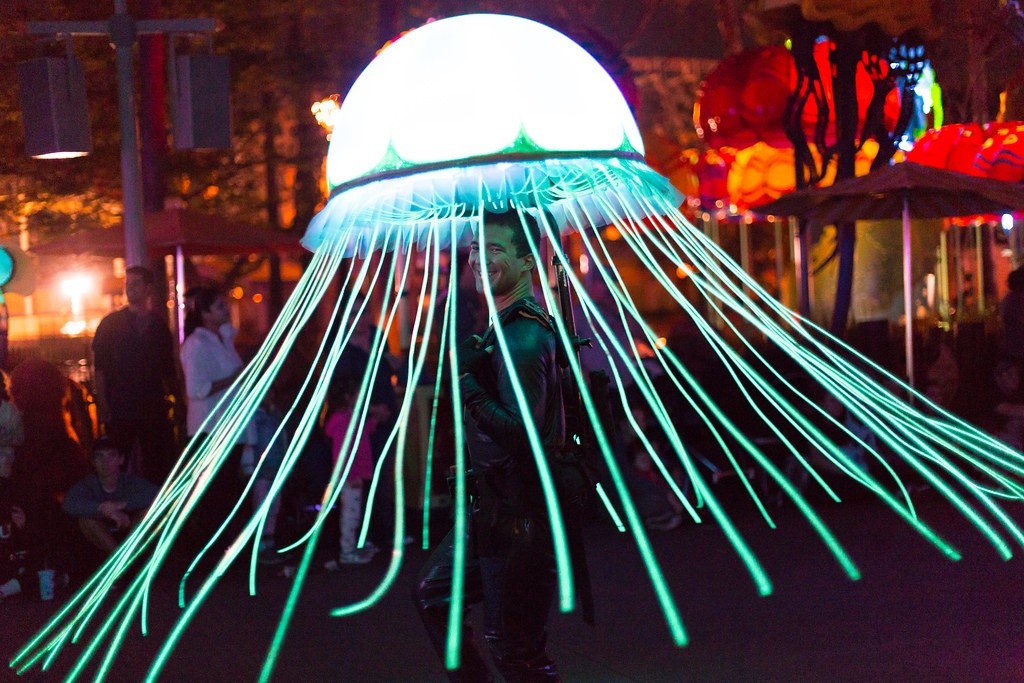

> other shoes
xmin=339 ymin=549 xmax=373 ymax=565
xmin=249 ymin=546 xmax=289 ymax=566
xmin=364 ymin=540 xmax=381 ymax=555
xmin=386 ymin=536 xmax=415 ymax=546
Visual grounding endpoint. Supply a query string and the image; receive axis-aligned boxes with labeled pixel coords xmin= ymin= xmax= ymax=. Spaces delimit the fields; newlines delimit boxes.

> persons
xmin=411 ymin=208 xmax=571 ymax=683
xmin=0 ymin=266 xmax=1024 ymax=604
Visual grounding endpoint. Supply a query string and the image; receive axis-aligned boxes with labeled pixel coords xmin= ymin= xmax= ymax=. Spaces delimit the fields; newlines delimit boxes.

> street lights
xmin=0 ymin=0 xmax=231 ymax=308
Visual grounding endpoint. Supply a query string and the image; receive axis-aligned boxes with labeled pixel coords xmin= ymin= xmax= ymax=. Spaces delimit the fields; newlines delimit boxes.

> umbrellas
xmin=29 ymin=209 xmax=307 ymax=352
xmin=749 ymin=161 xmax=1024 ymax=412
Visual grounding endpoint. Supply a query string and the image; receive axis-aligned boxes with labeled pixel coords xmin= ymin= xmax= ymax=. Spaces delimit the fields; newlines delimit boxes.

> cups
xmin=38 ymin=570 xmax=54 ymax=601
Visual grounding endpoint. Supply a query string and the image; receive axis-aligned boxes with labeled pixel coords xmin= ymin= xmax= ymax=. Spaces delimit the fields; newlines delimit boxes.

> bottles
xmin=17 ymin=550 xmax=34 ymax=582
